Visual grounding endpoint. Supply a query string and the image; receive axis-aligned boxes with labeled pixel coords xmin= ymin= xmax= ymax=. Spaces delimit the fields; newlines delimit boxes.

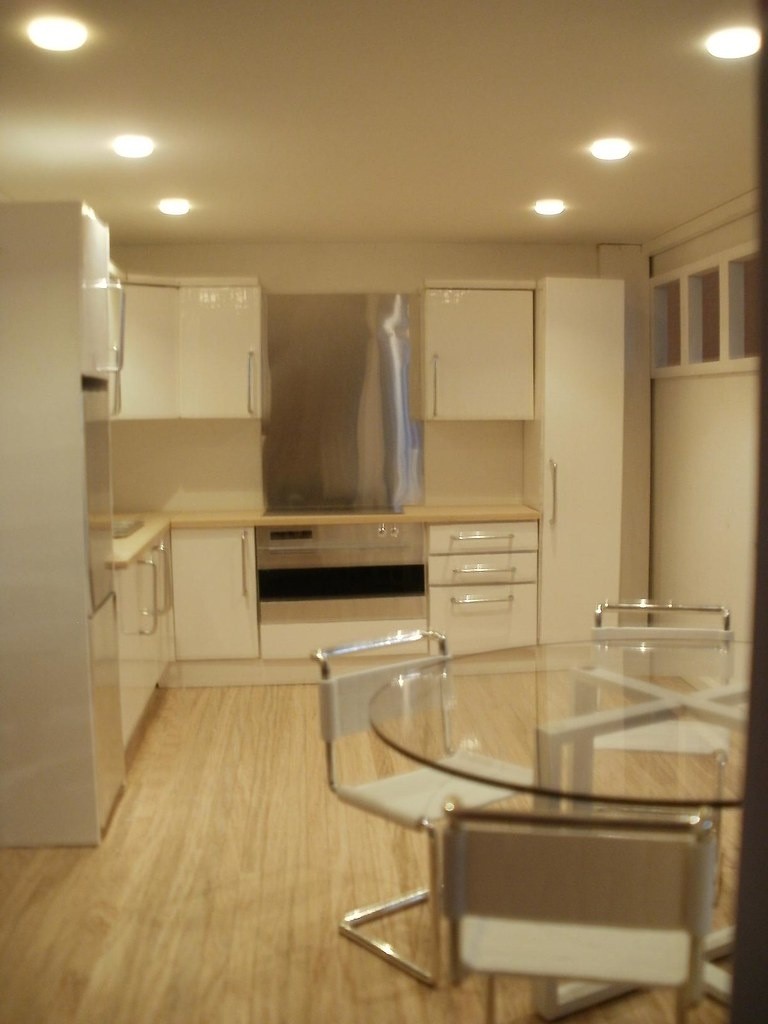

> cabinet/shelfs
xmin=169 ymin=527 xmax=261 ymax=661
xmin=404 ymin=278 xmax=535 ymax=422
xmin=114 ymin=532 xmax=175 ymax=771
xmin=111 ymin=273 xmax=262 ymax=419
xmin=425 ymin=520 xmax=539 ymax=657
xmin=523 ymin=276 xmax=627 ymax=643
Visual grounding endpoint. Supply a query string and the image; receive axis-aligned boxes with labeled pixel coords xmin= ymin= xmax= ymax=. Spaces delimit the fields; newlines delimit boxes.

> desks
xmin=368 ymin=636 xmax=751 ymax=1024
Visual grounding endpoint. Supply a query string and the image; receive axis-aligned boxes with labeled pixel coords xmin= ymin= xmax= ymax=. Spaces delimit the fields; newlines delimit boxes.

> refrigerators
xmin=0 ymin=376 xmax=127 ymax=849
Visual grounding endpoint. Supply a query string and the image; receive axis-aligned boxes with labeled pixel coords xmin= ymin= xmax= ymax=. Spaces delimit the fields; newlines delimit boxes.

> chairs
xmin=594 ymin=600 xmax=733 ymax=904
xmin=310 ymin=631 xmax=534 ymax=990
xmin=441 ymin=792 xmax=725 ymax=1024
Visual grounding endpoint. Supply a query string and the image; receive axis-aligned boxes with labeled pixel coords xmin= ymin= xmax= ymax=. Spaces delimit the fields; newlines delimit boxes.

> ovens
xmin=256 ymin=523 xmax=429 ymax=660
